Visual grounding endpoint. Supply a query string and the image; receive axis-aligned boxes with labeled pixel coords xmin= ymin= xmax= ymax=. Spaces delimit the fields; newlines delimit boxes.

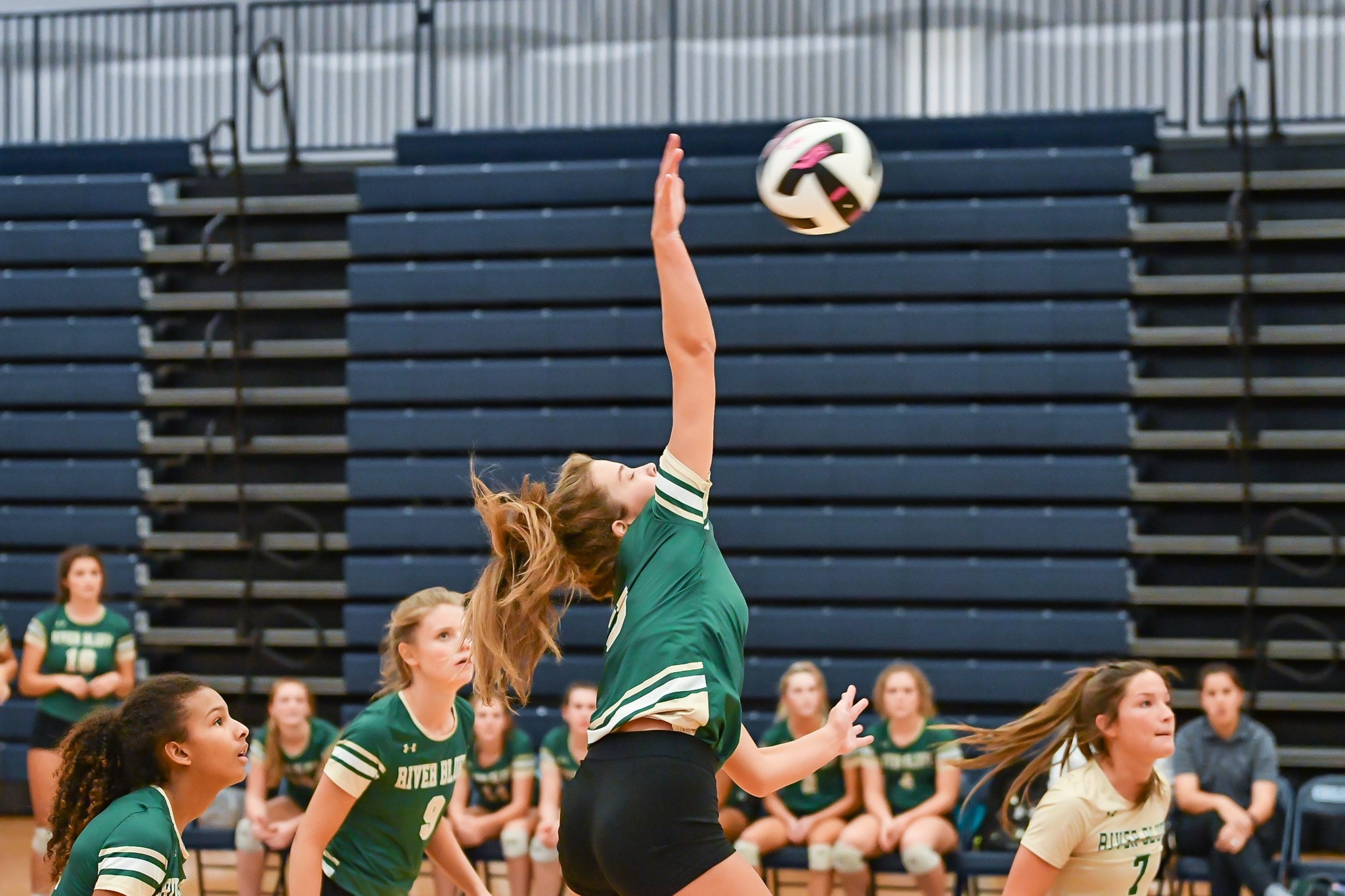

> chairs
xmin=176 ymin=769 xmax=1345 ymax=896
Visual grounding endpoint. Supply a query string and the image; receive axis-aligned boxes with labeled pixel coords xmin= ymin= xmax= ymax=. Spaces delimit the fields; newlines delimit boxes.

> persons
xmin=452 ymin=129 xmax=873 ymax=896
xmin=233 ymin=679 xmax=339 ymax=896
xmin=38 ymin=673 xmax=249 ymax=896
xmin=1164 ymin=662 xmax=1295 ymax=896
xmin=714 ymin=769 xmax=763 ymax=845
xmin=18 ymin=545 xmax=138 ymax=896
xmin=286 ymin=586 xmax=495 ymax=896
xmin=528 ymin=680 xmax=600 ymax=895
xmin=833 ymin=661 xmax=964 ymax=896
xmin=427 ymin=689 xmax=539 ymax=895
xmin=0 ymin=620 xmax=19 ymax=716
xmin=918 ymin=660 xmax=1176 ymax=896
xmin=732 ymin=661 xmax=863 ymax=896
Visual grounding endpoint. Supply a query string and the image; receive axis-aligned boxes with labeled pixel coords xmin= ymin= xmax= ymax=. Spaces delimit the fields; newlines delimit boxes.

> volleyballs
xmin=754 ymin=116 xmax=883 ymax=236
